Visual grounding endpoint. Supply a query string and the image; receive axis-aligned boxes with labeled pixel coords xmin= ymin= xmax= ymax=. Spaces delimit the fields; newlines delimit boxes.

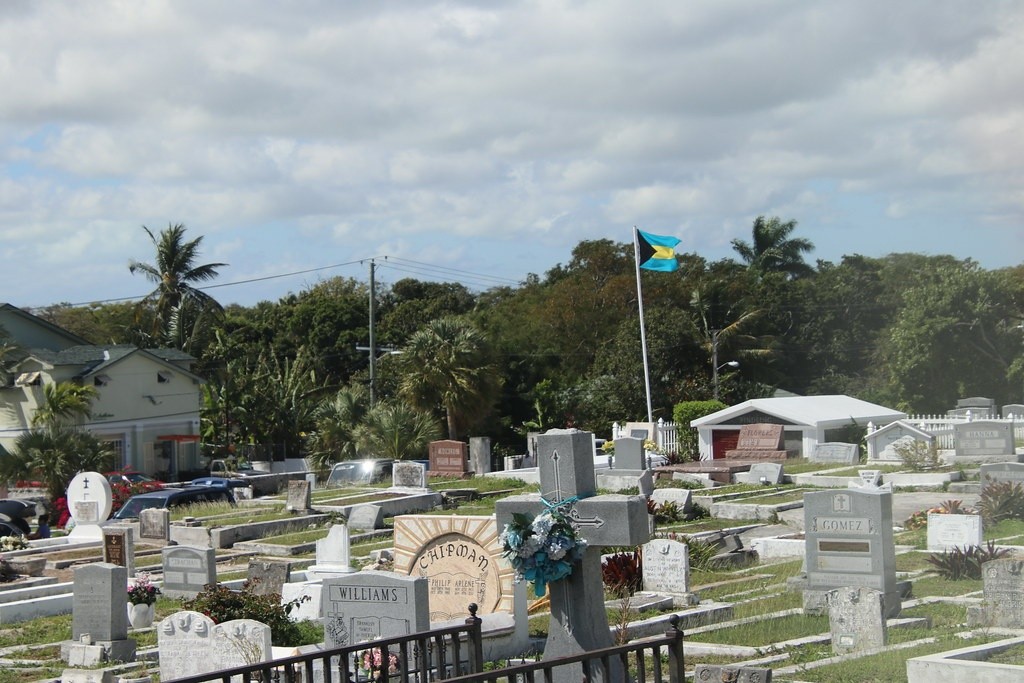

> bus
xmin=101 ymin=459 xmax=269 ymax=527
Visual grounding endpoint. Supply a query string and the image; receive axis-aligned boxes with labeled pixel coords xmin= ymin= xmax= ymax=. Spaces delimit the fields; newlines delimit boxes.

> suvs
xmin=325 ymin=458 xmax=400 ymax=490
xmin=0 ymin=496 xmax=51 ymax=545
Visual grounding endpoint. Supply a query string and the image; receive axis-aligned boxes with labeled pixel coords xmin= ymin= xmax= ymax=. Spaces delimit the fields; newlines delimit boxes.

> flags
xmin=637 ymin=229 xmax=682 ymax=273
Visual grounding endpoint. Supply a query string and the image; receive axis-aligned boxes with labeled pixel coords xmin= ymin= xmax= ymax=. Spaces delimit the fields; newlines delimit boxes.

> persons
xmin=27 ymin=512 xmax=51 ymax=540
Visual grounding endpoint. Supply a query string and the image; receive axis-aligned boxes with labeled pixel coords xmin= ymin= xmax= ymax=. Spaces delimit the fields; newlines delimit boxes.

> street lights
xmin=712 ymin=345 xmax=740 ymax=400
xmin=369 ymin=350 xmax=404 ymax=410
